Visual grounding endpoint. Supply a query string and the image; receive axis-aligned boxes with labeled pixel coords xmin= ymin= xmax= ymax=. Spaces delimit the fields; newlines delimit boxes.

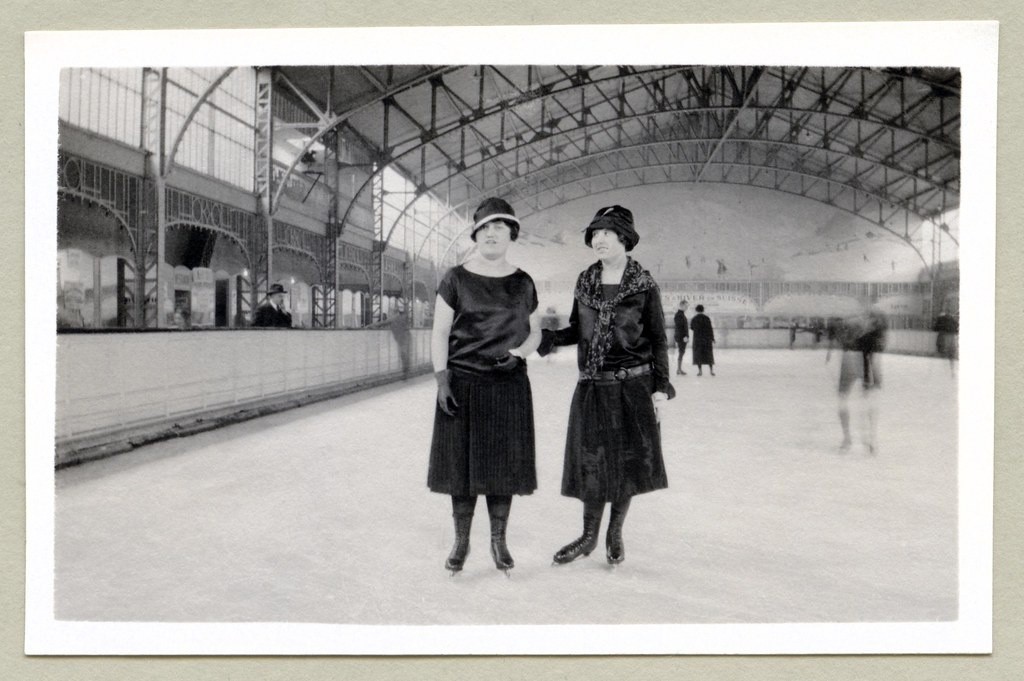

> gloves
xmin=434 ymin=369 xmax=460 ymax=418
xmin=655 ymin=378 xmax=676 ymax=400
xmin=494 ymin=351 xmax=519 ymax=371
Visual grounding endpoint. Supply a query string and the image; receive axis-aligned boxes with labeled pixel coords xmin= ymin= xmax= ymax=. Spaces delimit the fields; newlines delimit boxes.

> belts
xmin=577 ymin=361 xmax=655 ymax=381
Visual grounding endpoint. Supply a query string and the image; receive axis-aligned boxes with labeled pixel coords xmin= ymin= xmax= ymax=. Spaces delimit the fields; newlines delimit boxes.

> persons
xmin=690 ymin=304 xmax=717 ymax=376
xmin=788 ymin=299 xmax=889 ymax=454
xmin=250 ymin=283 xmax=293 ymax=328
xmin=536 ymin=205 xmax=676 ymax=563
xmin=674 ymin=299 xmax=690 ymax=375
xmin=427 ymin=197 xmax=543 ymax=571
xmin=932 ymin=306 xmax=958 ymax=377
xmin=539 ymin=308 xmax=560 ymax=361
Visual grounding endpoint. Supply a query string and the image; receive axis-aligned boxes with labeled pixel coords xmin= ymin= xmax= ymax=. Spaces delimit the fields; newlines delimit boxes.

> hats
xmin=471 ymin=196 xmax=521 ymax=242
xmin=267 ymin=284 xmax=287 ymax=295
xmin=584 ymin=204 xmax=640 ymax=251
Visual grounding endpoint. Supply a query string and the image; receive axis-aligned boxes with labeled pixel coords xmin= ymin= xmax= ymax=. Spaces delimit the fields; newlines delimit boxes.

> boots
xmin=489 ymin=516 xmax=515 ymax=570
xmin=444 ymin=511 xmax=473 ymax=572
xmin=604 ymin=508 xmax=625 ymax=565
xmin=553 ymin=513 xmax=602 ymax=564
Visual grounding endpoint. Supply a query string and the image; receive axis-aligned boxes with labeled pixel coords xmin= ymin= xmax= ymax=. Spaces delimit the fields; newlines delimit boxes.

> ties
xmin=277 ymin=304 xmax=280 ymax=312
